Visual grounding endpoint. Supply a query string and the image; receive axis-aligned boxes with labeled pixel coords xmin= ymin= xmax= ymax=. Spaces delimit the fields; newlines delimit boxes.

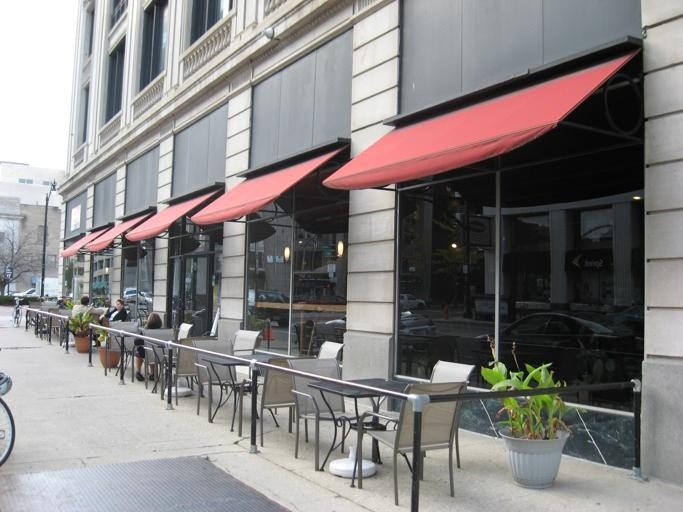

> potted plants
xmin=480 ymin=332 xmax=573 ymax=490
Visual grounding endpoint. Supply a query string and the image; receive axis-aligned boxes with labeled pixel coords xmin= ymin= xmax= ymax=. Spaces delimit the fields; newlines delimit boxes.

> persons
xmin=71 ymin=296 xmax=90 ymax=321
xmin=92 ymin=299 xmax=128 ymax=349
xmin=134 ymin=311 xmax=162 ymax=381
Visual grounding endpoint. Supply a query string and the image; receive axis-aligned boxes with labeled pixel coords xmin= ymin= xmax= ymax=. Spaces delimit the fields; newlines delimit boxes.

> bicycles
xmin=0 ymin=373 xmax=14 ymax=467
xmin=11 ymin=297 xmax=25 ymax=328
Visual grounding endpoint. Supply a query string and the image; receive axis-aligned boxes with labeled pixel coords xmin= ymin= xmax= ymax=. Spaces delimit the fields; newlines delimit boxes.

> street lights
xmin=39 ymin=179 xmax=56 ymax=298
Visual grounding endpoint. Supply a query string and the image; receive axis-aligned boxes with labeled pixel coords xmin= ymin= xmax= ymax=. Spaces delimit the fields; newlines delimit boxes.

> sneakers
xmin=135 ymin=373 xmax=144 ymax=381
xmin=149 ymin=374 xmax=158 ymax=380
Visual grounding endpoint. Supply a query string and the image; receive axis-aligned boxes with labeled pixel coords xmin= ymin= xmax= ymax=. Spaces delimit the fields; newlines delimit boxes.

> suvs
xmin=244 ymin=287 xmax=291 ymax=328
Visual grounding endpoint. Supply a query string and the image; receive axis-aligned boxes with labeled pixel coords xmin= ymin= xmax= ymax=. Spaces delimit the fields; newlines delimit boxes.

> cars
xmin=398 ymin=291 xmax=429 ymax=310
xmin=461 ymin=305 xmax=654 ymax=407
xmin=88 ymin=285 xmax=138 ymax=303
xmin=183 ymin=308 xmax=210 ymax=338
xmin=322 ymin=308 xmax=436 ymax=361
xmin=12 ymin=289 xmax=37 ymax=297
xmin=602 ymin=306 xmax=642 ymax=346
xmin=138 ymin=292 xmax=152 ymax=305
xmin=291 ymin=295 xmax=346 ymax=328
